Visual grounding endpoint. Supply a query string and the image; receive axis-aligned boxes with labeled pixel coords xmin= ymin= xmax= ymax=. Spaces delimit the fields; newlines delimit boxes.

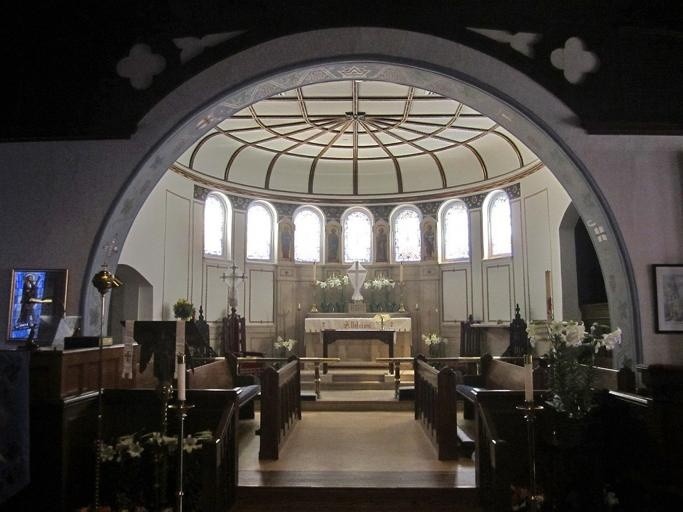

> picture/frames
xmin=652 ymin=262 xmax=683 ymax=333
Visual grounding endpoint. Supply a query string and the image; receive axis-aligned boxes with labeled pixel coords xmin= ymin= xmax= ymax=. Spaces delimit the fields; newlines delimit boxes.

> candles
xmin=544 ymin=268 xmax=554 ymax=322
xmin=523 ymin=353 xmax=534 ymax=403
xmin=176 ymin=352 xmax=187 ymax=401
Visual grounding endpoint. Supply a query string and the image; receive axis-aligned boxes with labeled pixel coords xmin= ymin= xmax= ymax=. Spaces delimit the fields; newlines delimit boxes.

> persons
xmin=15 ymin=274 xmax=37 ymax=329
